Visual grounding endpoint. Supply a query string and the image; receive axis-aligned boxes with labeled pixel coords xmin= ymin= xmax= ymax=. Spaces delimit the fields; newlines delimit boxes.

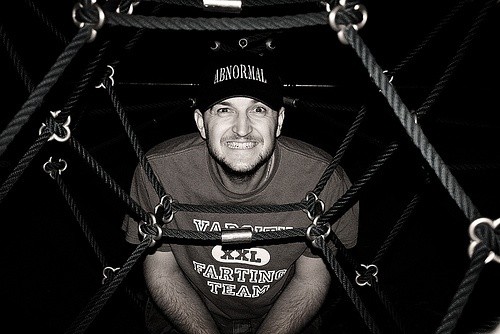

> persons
xmin=122 ymin=52 xmax=361 ymax=334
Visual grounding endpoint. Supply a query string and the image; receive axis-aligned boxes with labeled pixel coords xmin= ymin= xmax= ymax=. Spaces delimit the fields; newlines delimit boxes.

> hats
xmin=195 ymin=49 xmax=283 ymax=112
